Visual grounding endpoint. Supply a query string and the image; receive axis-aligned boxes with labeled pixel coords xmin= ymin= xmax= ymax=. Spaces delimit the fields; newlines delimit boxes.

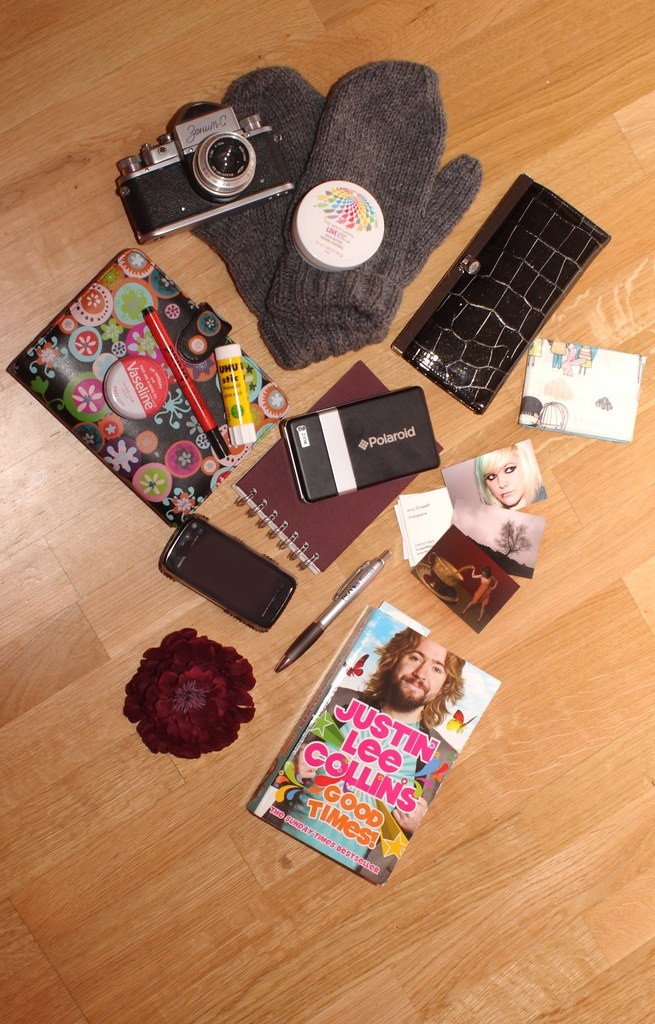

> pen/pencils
xmin=272 ymin=548 xmax=395 ymax=673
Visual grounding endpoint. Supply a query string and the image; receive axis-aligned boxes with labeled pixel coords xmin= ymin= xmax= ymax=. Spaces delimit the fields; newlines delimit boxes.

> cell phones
xmin=158 ymin=517 xmax=298 ymax=632
xmin=284 ymin=386 xmax=440 ymax=504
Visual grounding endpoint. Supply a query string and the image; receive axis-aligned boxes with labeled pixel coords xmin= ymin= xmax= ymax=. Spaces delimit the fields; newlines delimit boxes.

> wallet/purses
xmin=401 ymin=182 xmax=611 ymax=416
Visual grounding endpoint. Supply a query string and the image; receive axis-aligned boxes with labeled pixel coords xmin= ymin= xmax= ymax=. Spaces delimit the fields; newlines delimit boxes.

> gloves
xmin=187 ymin=66 xmax=390 ymax=371
xmin=263 ymin=57 xmax=484 ymax=334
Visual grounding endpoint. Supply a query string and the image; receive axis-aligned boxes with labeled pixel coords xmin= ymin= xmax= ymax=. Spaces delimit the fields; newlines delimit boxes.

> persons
xmin=463 ymin=566 xmax=498 ymax=624
xmin=281 ymin=627 xmax=466 ymax=885
xmin=475 ymin=445 xmax=547 ymax=511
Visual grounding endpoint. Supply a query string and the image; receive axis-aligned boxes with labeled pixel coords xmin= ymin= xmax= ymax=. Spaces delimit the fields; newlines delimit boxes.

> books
xmin=231 ymin=360 xmax=444 ymax=574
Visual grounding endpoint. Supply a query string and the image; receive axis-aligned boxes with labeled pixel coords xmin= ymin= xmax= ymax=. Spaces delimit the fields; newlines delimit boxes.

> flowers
xmin=124 ymin=627 xmax=258 ymax=759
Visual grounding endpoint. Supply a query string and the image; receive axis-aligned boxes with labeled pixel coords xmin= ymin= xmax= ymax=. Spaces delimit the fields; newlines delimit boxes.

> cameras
xmin=117 ymin=106 xmax=295 ymax=245
